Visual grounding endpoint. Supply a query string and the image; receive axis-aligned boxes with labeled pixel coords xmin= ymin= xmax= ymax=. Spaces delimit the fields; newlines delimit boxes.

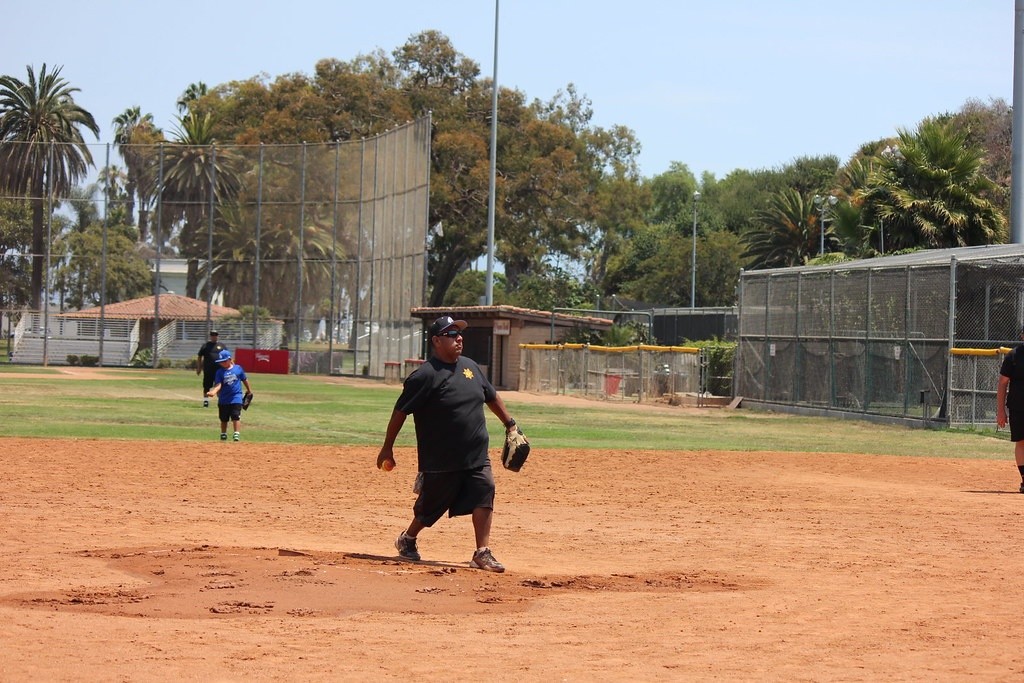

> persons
xmin=206 ymin=348 xmax=251 ymax=441
xmin=376 ymin=316 xmax=531 ymax=571
xmin=196 ymin=329 xmax=227 ymax=407
xmin=997 ymin=327 xmax=1024 ymax=493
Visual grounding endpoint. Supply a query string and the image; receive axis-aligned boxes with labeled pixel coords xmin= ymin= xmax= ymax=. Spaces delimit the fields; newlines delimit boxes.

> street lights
xmin=814 ymin=194 xmax=839 ymax=258
xmin=692 ymin=190 xmax=701 ymax=308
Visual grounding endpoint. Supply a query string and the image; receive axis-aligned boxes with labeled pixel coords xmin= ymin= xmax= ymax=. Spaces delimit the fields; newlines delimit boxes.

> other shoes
xmin=233 ymin=432 xmax=240 ymax=441
xmin=220 ymin=434 xmax=227 ymax=440
xmin=203 ymin=400 xmax=208 ymax=407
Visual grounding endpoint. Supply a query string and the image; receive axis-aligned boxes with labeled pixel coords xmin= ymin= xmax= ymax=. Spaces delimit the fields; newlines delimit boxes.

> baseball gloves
xmin=241 ymin=390 xmax=254 ymax=411
xmin=500 ymin=422 xmax=533 ymax=473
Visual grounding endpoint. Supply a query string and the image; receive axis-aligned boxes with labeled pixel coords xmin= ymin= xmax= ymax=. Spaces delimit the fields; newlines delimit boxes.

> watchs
xmin=505 ymin=420 xmax=516 ymax=429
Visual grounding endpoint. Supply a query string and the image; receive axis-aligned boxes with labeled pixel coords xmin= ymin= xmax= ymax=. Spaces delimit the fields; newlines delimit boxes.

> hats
xmin=215 ymin=350 xmax=232 ymax=363
xmin=210 ymin=329 xmax=218 ymax=336
xmin=429 ymin=316 xmax=468 ymax=337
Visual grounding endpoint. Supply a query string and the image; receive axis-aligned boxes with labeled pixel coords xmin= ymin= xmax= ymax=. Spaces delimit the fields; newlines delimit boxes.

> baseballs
xmin=382 ymin=460 xmax=394 ymax=473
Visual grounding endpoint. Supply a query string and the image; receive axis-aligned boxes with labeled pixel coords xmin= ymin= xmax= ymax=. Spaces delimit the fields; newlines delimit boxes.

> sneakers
xmin=470 ymin=548 xmax=505 ymax=573
xmin=394 ymin=529 xmax=421 ymax=560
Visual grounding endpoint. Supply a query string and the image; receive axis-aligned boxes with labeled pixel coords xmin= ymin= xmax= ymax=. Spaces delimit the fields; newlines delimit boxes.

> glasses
xmin=437 ymin=331 xmax=464 ymax=337
